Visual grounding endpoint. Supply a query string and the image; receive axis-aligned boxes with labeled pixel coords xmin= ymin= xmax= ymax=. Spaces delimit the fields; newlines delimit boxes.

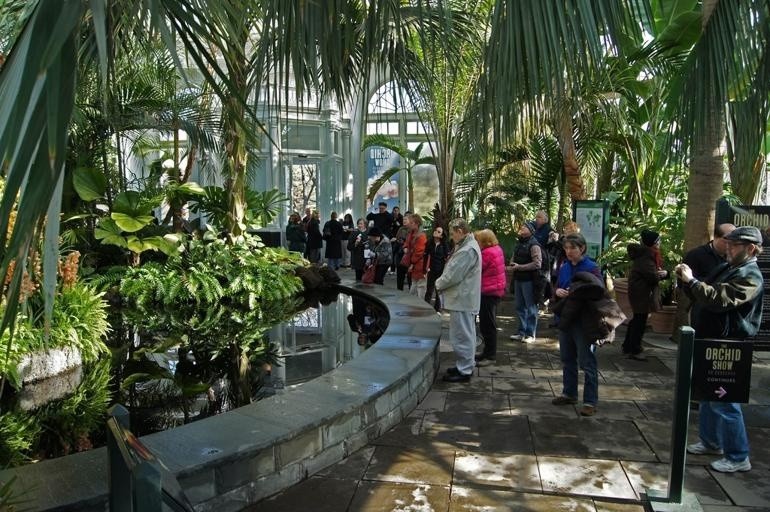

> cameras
xmin=676 ymin=268 xmax=682 ymax=277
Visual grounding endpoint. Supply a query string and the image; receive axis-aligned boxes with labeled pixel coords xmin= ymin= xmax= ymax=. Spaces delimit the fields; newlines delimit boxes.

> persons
xmin=625 ymin=228 xmax=671 ymax=363
xmin=287 ymin=201 xmax=448 ymax=317
xmin=531 ymin=211 xmax=581 ymax=328
xmin=347 ymin=296 xmax=389 ymax=346
xmin=510 ymin=221 xmax=546 ymax=344
xmin=432 ymin=217 xmax=483 ymax=381
xmin=675 ymin=226 xmax=764 ymax=473
xmin=554 ymin=231 xmax=609 ymax=415
xmin=681 ymin=220 xmax=733 ymax=337
xmin=473 ymin=229 xmax=507 ymax=366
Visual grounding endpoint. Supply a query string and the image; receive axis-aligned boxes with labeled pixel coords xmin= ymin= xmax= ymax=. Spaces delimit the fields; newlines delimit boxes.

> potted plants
xmin=651 ymin=210 xmax=685 ymax=333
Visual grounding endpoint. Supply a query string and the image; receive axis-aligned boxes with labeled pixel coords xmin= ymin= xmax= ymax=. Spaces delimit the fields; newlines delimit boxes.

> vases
xmin=613 ymin=277 xmax=651 ymax=327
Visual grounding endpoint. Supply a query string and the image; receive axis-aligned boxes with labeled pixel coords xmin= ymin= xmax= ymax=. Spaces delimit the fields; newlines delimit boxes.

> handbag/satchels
xmin=361 ymin=252 xmax=379 ymax=283
xmin=399 ymin=234 xmax=411 ymax=268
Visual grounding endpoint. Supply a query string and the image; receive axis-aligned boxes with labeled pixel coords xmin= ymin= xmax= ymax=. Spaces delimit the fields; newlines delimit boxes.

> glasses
xmin=434 ymin=229 xmax=442 ymax=234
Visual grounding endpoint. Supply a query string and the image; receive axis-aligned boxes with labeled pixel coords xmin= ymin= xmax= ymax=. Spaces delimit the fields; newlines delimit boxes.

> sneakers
xmin=521 ymin=335 xmax=536 ymax=344
xmin=510 ymin=333 xmax=525 ymax=341
xmin=475 ymin=353 xmax=497 ymax=367
xmin=442 ymin=366 xmax=474 ymax=382
xmin=577 ymin=405 xmax=598 ymax=417
xmin=620 ymin=344 xmax=648 ymax=361
xmin=709 ymin=455 xmax=753 ymax=474
xmin=686 ymin=442 xmax=724 ymax=456
xmin=552 ymin=395 xmax=578 ymax=405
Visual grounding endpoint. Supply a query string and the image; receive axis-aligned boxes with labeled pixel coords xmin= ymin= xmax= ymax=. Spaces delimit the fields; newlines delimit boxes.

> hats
xmin=640 ymin=229 xmax=660 ymax=247
xmin=722 ymin=225 xmax=763 ymax=246
xmin=379 ymin=202 xmax=387 ymax=207
xmin=523 ymin=221 xmax=538 ymax=237
